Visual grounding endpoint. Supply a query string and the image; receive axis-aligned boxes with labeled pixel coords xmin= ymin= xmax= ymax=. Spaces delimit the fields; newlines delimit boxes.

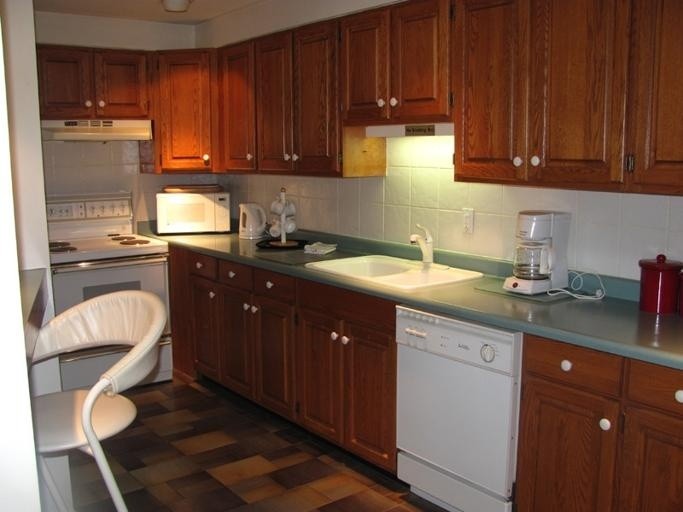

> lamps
xmin=161 ymin=0 xmax=192 ymax=11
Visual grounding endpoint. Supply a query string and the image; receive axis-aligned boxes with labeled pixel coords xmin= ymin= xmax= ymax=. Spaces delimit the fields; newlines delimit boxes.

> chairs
xmin=28 ymin=286 xmax=171 ymax=512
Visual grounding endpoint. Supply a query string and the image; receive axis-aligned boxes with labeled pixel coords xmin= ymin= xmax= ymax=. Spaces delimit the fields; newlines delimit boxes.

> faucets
xmin=408 ymin=223 xmax=435 ymax=265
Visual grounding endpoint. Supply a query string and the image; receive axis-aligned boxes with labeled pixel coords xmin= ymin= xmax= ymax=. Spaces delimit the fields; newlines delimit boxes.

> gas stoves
xmin=46 ymin=220 xmax=167 ymax=264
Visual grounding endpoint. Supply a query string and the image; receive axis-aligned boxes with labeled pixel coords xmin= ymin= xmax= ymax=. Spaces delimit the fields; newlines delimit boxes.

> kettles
xmin=238 ymin=203 xmax=266 ymax=240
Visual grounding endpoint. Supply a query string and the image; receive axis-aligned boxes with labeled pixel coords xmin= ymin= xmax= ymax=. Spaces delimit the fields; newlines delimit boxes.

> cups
xmin=268 ymin=196 xmax=296 ymax=237
xmin=635 ymin=255 xmax=681 ymax=313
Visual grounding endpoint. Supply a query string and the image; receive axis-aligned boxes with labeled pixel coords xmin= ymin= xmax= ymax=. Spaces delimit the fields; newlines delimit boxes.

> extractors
xmin=39 ymin=120 xmax=155 ymax=142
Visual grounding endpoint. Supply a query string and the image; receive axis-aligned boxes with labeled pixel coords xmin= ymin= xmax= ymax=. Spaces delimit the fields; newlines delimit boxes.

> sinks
xmin=369 ymin=267 xmax=485 ymax=291
xmin=304 ymin=254 xmax=413 ymax=280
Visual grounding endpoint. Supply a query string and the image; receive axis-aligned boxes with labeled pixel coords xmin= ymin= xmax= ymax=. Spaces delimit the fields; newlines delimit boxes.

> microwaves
xmin=153 ymin=193 xmax=231 ymax=235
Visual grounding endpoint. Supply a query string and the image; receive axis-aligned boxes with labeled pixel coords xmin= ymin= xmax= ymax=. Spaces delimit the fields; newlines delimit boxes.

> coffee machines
xmin=502 ymin=209 xmax=572 ymax=296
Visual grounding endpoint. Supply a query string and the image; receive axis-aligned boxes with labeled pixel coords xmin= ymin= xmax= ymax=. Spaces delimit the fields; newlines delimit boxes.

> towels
xmin=303 ymin=240 xmax=338 ymax=256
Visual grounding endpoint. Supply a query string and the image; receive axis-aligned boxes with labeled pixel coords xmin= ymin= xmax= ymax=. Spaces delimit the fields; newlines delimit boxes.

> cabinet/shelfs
xmin=166 ymin=244 xmax=221 ymax=389
xmin=513 ymin=336 xmax=624 ymax=512
xmin=138 ymin=48 xmax=218 ymax=174
xmin=618 ymin=1 xmax=681 ymax=198
xmin=337 ymin=0 xmax=451 ymax=126
xmin=614 ymin=353 xmax=682 ymax=512
xmin=251 ymin=17 xmax=386 ymax=178
xmin=443 ymin=0 xmax=626 ymax=192
xmin=218 ymin=28 xmax=256 ymax=174
xmin=218 ymin=260 xmax=297 ymax=427
xmin=296 ymin=279 xmax=396 ymax=475
xmin=35 ymin=43 xmax=149 ymax=120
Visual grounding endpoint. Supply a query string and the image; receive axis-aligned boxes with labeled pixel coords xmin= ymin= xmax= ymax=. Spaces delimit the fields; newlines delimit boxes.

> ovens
xmin=50 ymin=254 xmax=172 ymax=357
